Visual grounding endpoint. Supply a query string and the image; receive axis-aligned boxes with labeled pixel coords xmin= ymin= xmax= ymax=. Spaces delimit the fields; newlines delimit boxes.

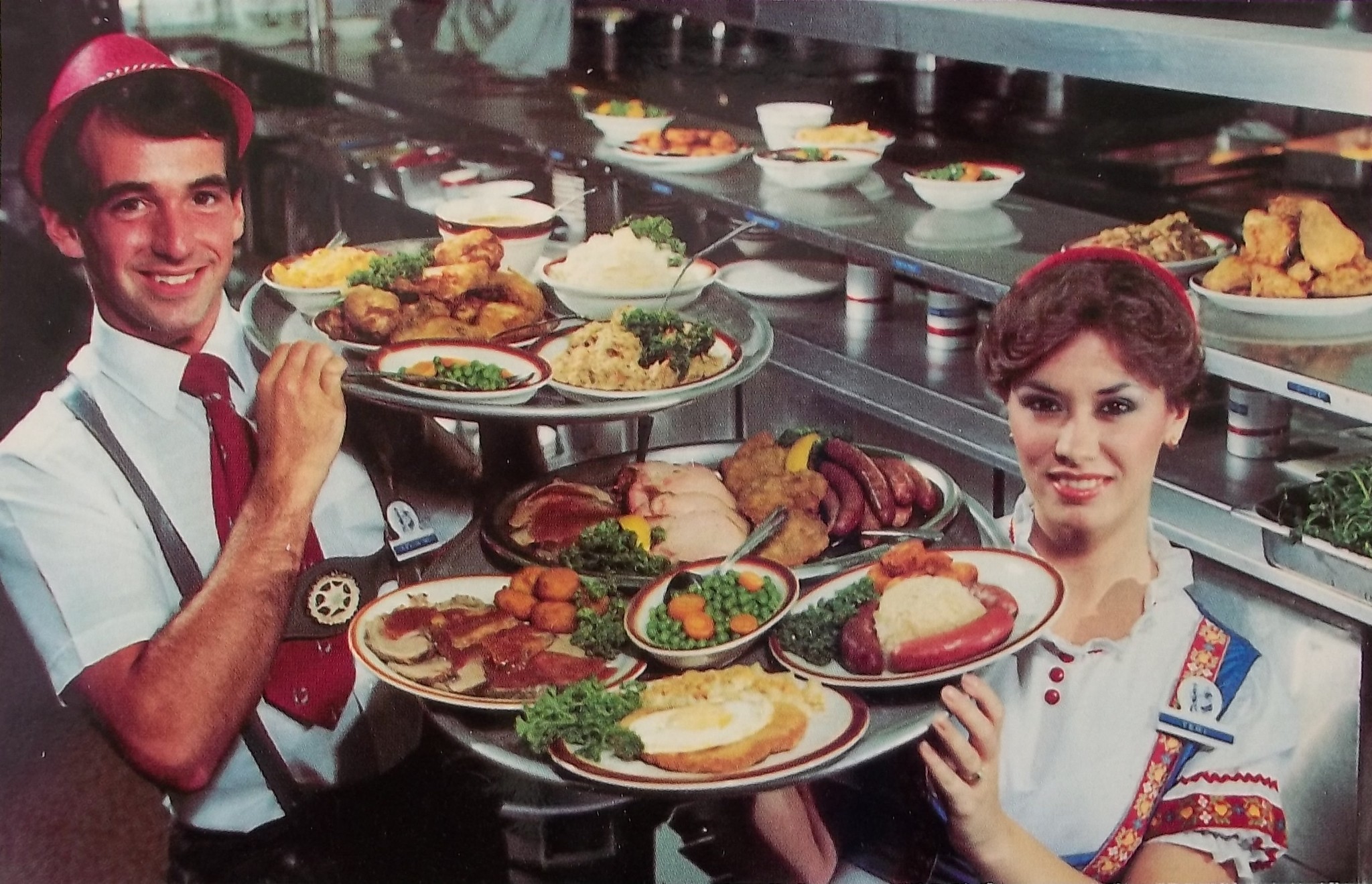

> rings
xmin=962 ymin=760 xmax=985 ymax=783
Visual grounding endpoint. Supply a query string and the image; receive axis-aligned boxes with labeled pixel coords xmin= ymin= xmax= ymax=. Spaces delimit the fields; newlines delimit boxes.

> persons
xmin=738 ymin=242 xmax=1292 ymax=884
xmin=0 ymin=27 xmax=557 ymax=884
xmin=431 ymin=0 xmax=575 ymax=83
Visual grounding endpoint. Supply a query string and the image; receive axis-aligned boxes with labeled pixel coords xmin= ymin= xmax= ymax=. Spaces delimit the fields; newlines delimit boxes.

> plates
xmin=716 ymin=256 xmax=844 ymax=299
xmin=903 ymin=165 xmax=1027 ymax=209
xmin=313 ymin=296 xmax=561 ymax=356
xmin=469 ymin=435 xmax=963 ymax=578
xmin=532 ymin=318 xmax=744 ymax=404
xmin=616 ymin=137 xmax=753 ymax=175
xmin=540 ymin=670 xmax=871 ymax=790
xmin=767 ymin=548 xmax=1066 ymax=688
xmin=1059 ymin=224 xmax=1240 ymax=277
xmin=371 ymin=340 xmax=554 ymax=410
xmin=552 ymin=165 xmax=696 ymax=233
xmin=345 ymin=572 xmax=648 ymax=712
xmin=1186 ymin=266 xmax=1372 ymax=320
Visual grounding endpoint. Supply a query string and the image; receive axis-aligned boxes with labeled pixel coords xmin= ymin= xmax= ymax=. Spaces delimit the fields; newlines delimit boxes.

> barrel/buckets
xmin=1226 ymin=378 xmax=1292 ymax=458
xmin=924 ymin=287 xmax=980 ymax=348
xmin=844 ymin=259 xmax=898 ymax=301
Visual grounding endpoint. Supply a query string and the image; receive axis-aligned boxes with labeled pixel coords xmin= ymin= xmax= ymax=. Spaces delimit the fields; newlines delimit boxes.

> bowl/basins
xmin=260 ymin=246 xmax=394 ymax=316
xmin=791 ymin=133 xmax=898 ymax=153
xmin=729 ymin=216 xmax=791 ymax=259
xmin=428 ymin=152 xmax=554 ymax=295
xmin=627 ymin=552 xmax=801 ymax=670
xmin=536 ymin=245 xmax=718 ymax=313
xmin=752 ymin=146 xmax=883 ymax=192
xmin=755 ymin=100 xmax=835 ymax=150
xmin=582 ymin=104 xmax=677 ymax=141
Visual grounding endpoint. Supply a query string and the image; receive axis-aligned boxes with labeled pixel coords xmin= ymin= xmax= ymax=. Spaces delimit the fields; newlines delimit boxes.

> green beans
xmin=395 ymin=356 xmax=508 ymax=393
xmin=645 ymin=569 xmax=782 ymax=652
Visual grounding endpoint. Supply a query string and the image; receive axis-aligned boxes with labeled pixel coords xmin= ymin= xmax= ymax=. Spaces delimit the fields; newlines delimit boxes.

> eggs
xmin=625 ymin=699 xmax=773 ymax=755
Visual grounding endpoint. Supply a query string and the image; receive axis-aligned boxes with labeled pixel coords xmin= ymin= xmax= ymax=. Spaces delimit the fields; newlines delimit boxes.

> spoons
xmin=662 ymin=505 xmax=789 ymax=604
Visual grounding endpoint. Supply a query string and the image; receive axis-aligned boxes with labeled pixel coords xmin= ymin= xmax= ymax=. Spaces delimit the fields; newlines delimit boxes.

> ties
xmin=179 ymin=353 xmax=357 ymax=730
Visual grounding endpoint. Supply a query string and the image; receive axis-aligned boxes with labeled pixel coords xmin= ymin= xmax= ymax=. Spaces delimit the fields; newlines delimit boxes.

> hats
xmin=19 ymin=32 xmax=254 ymax=204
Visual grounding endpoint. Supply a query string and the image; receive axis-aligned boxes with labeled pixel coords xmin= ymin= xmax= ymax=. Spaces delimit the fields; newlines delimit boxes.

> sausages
xmin=817 ymin=438 xmax=938 ymax=548
xmin=838 ymin=581 xmax=1018 ymax=676
